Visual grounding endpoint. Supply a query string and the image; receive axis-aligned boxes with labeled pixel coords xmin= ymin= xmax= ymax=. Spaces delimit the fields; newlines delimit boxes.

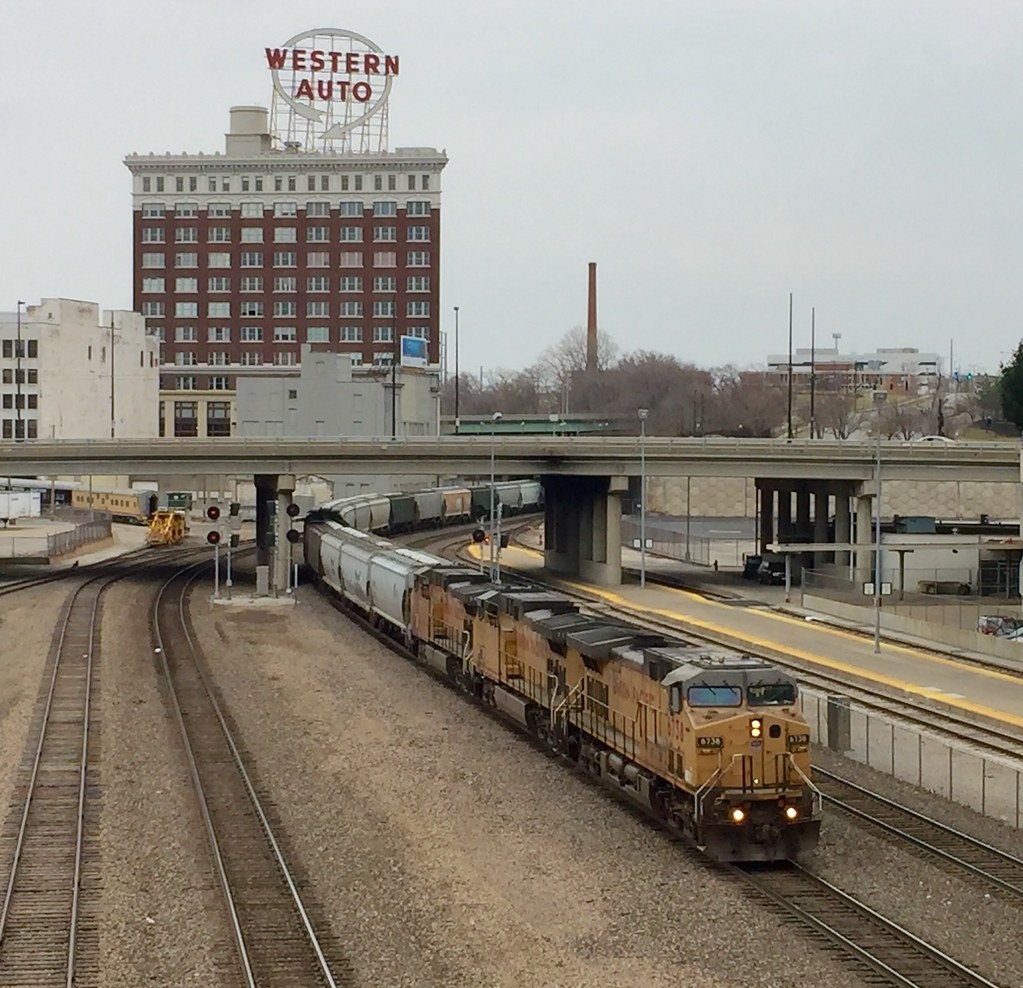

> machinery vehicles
xmin=145 ymin=510 xmax=187 ymax=547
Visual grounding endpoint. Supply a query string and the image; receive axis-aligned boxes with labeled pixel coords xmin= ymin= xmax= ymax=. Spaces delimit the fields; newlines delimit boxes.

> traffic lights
xmin=286 ymin=529 xmax=300 ymax=543
xmin=207 ymin=506 xmax=219 ymax=520
xmin=207 ymin=531 xmax=220 ymax=544
xmin=287 ymin=503 xmax=300 ymax=517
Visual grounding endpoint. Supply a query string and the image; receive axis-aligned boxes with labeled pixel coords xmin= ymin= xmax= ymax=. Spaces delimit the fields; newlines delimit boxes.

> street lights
xmin=453 ymin=305 xmax=459 ymax=436
xmin=489 ymin=410 xmax=503 ymax=580
xmin=15 ymin=299 xmax=26 ymax=442
xmin=637 ymin=408 xmax=650 ymax=589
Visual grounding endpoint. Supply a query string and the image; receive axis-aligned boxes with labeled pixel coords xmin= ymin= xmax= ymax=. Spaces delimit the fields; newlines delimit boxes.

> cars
xmin=916 ymin=436 xmax=955 ymax=443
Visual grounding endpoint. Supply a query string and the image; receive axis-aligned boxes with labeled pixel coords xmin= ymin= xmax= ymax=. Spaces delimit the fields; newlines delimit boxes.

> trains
xmin=302 ymin=473 xmax=826 ymax=871
xmin=0 ymin=475 xmax=159 ymax=526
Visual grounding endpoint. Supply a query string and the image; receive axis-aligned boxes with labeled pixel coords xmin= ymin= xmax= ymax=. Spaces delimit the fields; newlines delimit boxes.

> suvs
xmin=757 ymin=561 xmax=792 ymax=585
